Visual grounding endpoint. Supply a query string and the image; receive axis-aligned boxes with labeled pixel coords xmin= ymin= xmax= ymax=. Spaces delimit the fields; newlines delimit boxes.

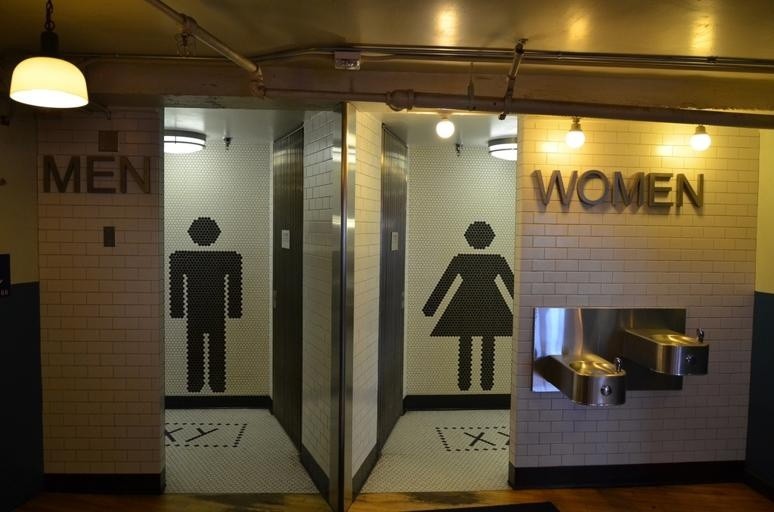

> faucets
xmin=696 ymin=328 xmax=704 ymax=341
xmin=615 ymin=358 xmax=621 ymax=372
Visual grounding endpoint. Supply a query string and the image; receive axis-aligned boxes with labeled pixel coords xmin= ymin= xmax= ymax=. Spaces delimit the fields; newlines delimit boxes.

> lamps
xmin=164 ymin=128 xmax=205 ymax=155
xmin=488 ymin=136 xmax=517 ymax=162
xmin=10 ymin=0 xmax=88 ymax=109
xmin=690 ymin=124 xmax=711 ymax=152
xmin=566 ymin=115 xmax=585 ymax=151
xmin=435 ymin=110 xmax=454 ymax=140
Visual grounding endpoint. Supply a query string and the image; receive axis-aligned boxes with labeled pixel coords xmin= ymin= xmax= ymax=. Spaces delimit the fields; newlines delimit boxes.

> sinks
xmin=649 ymin=335 xmax=697 ymax=345
xmin=569 ymin=361 xmax=618 ymax=375
